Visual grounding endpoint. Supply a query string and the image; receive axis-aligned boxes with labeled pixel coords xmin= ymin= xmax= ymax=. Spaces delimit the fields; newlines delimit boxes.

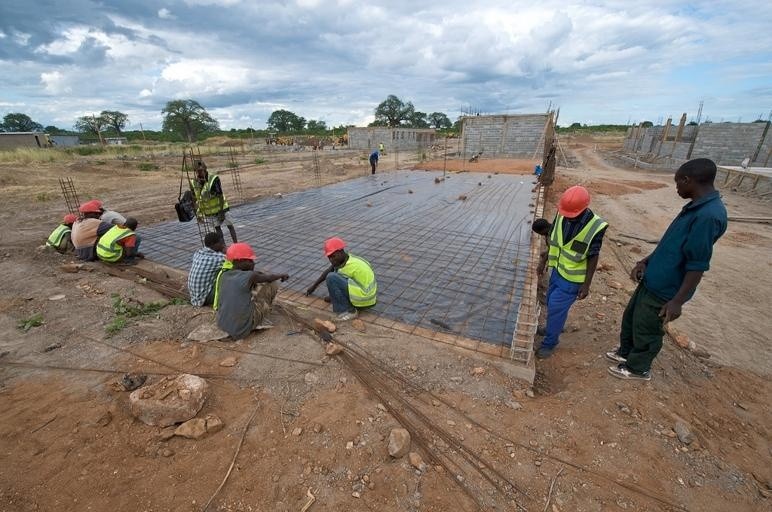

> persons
xmin=370 ymin=150 xmax=380 ymax=174
xmin=303 ymin=237 xmax=378 ymax=321
xmin=95 ymin=216 xmax=144 ymax=265
xmin=535 ymin=185 xmax=610 ymax=359
xmin=45 ymin=214 xmax=77 ymax=254
xmin=187 ymin=233 xmax=228 ymax=307
xmin=71 ymin=203 xmax=115 ymax=261
xmin=531 ymin=219 xmax=551 ymax=235
xmin=606 ymin=158 xmax=728 ymax=380
xmin=88 ymin=200 xmax=127 ymax=225
xmin=191 ymin=162 xmax=237 ymax=253
xmin=378 ymin=142 xmax=384 ymax=156
xmin=216 ymin=243 xmax=289 ymax=341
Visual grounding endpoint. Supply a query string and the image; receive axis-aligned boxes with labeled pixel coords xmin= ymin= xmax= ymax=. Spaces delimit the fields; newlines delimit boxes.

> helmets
xmin=324 ymin=238 xmax=348 ymax=257
xmin=557 ymin=186 xmax=590 ymax=218
xmin=64 ymin=200 xmax=104 ymax=225
xmin=226 ymin=243 xmax=258 ymax=261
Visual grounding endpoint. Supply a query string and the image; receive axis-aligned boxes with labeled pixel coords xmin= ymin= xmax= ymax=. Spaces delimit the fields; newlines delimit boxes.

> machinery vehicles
xmin=265 ymin=133 xmax=293 ymax=146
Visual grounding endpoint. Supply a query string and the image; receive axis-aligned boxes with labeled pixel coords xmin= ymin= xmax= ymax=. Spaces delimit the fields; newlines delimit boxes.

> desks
xmin=718 ymin=166 xmax=772 ymax=189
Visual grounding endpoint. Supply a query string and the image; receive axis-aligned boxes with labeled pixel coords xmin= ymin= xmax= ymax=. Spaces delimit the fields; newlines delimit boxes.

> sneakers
xmin=604 ymin=349 xmax=628 ymax=364
xmin=535 ymin=324 xmax=562 ymax=357
xmin=334 ymin=308 xmax=358 ymax=322
xmin=607 ymin=362 xmax=651 ymax=381
xmin=256 ymin=316 xmax=276 ymax=330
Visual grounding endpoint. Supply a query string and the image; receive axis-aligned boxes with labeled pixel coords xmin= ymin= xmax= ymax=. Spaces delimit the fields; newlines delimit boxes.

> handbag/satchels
xmin=175 ymin=191 xmax=199 ymax=223
